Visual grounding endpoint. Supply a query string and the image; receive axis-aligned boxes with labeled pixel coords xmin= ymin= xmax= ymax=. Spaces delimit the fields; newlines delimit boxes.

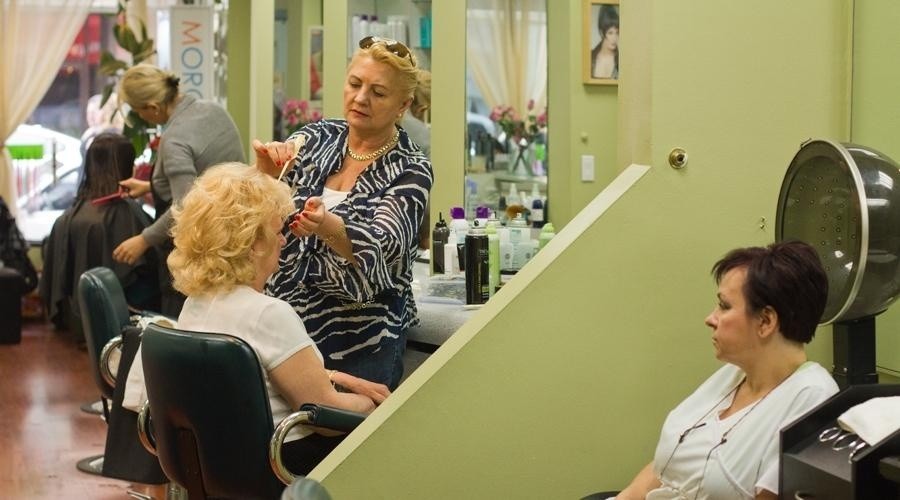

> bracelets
xmin=328 ymin=369 xmax=338 ymax=388
xmin=320 ymin=216 xmax=346 ymax=246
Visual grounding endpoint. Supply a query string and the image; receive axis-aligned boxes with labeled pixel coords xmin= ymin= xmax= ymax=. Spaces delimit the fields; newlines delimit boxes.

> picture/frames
xmin=581 ymin=0 xmax=620 ymax=86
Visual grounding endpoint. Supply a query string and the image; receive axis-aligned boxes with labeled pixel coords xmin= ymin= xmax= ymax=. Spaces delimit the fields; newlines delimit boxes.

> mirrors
xmin=347 ymin=1 xmax=433 ymax=250
xmin=462 ymin=0 xmax=550 ymax=229
xmin=272 ymin=1 xmax=324 ymax=142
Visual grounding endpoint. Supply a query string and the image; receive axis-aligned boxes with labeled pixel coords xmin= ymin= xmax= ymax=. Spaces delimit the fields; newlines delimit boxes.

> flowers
xmin=490 ymin=100 xmax=548 ymax=175
xmin=282 ymin=101 xmax=323 ymax=139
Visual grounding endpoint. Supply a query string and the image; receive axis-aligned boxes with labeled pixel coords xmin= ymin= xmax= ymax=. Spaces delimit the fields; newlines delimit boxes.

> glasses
xmin=359 ymin=36 xmax=414 ymax=65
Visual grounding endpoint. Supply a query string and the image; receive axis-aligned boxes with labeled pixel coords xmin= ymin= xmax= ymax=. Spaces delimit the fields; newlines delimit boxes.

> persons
xmin=398 ymin=68 xmax=432 ymax=161
xmin=71 ymin=132 xmax=138 ymax=206
xmin=590 ymin=4 xmax=620 ymax=79
xmin=167 ymin=159 xmax=392 ymax=477
xmin=602 ymin=238 xmax=841 ymax=500
xmin=111 ymin=63 xmax=248 ymax=320
xmin=247 ymin=34 xmax=436 ymax=457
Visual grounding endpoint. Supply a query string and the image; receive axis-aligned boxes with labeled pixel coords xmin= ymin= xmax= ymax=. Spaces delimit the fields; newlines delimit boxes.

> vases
xmin=506 ymin=135 xmax=533 ymax=177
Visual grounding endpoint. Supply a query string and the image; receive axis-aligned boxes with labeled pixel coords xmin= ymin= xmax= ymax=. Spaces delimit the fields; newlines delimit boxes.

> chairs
xmin=142 ymin=322 xmax=369 ymax=500
xmin=76 ymin=266 xmax=142 ymax=475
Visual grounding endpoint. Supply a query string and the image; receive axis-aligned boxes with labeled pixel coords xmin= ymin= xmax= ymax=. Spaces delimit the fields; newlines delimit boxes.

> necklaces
xmin=346 ymin=129 xmax=401 ymax=161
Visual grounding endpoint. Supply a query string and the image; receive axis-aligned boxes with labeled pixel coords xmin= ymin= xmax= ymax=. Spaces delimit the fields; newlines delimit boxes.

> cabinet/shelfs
xmin=776 ymin=382 xmax=899 ymax=500
